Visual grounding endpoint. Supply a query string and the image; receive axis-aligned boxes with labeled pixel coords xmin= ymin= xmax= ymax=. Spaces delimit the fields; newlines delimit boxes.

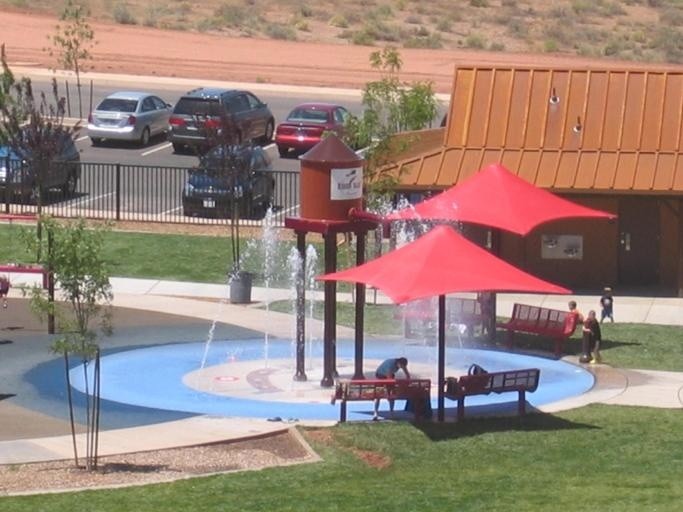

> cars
xmin=274 ymin=102 xmax=368 ymax=155
xmin=87 ymin=92 xmax=176 ymax=148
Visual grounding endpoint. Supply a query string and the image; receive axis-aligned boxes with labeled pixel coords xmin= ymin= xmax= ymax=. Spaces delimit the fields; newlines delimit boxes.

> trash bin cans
xmin=230 ymin=271 xmax=252 ymax=303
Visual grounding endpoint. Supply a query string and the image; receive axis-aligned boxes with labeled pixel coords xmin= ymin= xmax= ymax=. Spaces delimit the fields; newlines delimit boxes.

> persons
xmin=599 ymin=287 xmax=614 ymax=324
xmin=568 ymin=300 xmax=584 ymax=323
xmin=372 ymin=358 xmax=410 ymax=420
xmin=580 ymin=310 xmax=601 ymax=364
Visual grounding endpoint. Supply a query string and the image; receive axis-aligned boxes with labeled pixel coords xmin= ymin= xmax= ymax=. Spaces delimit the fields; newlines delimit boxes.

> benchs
xmin=394 ymin=296 xmax=483 ymax=345
xmin=332 ymin=376 xmax=432 ymax=426
xmin=445 ymin=367 xmax=541 ymax=420
xmin=498 ymin=302 xmax=579 ymax=358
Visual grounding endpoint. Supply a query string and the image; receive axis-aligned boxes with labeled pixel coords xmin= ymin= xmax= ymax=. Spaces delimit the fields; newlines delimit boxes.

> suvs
xmin=166 ymin=89 xmax=276 ymax=150
xmin=182 ymin=144 xmax=279 ymax=218
xmin=0 ymin=123 xmax=81 ymax=204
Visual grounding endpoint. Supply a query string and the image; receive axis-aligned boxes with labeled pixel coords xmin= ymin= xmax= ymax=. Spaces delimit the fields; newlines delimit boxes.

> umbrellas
xmin=386 ymin=161 xmax=619 ymax=343
xmin=314 ymin=224 xmax=573 ymax=422
